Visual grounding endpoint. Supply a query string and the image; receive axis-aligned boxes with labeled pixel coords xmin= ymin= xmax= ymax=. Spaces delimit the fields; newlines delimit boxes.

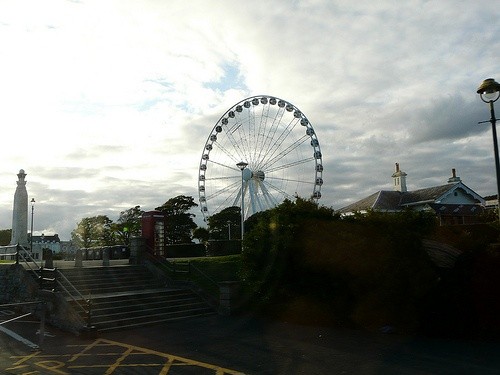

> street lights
xmin=30 ymin=198 xmax=35 ymax=256
xmin=477 ymin=79 xmax=500 ymax=225
